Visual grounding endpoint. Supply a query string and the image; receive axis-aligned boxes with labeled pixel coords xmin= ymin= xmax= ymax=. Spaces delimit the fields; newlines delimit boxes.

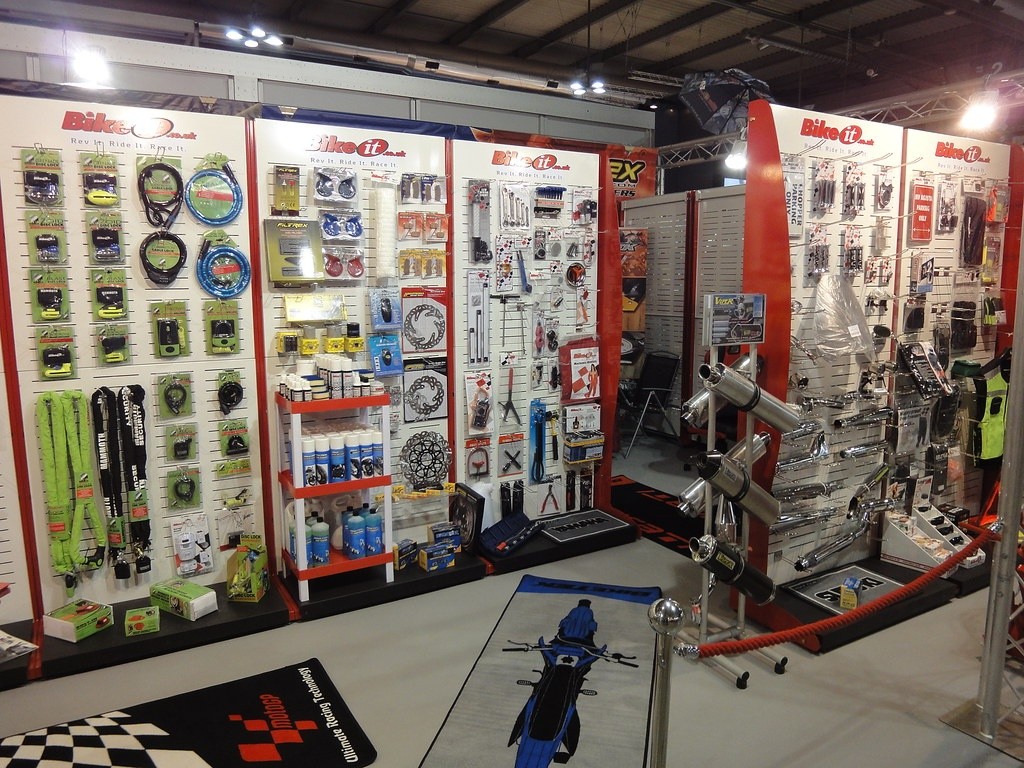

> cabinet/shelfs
xmin=275 ymin=389 xmax=397 ymax=602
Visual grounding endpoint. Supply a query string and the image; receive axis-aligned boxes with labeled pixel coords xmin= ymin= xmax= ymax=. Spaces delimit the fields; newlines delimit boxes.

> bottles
xmin=279 ymin=353 xmax=384 ymax=568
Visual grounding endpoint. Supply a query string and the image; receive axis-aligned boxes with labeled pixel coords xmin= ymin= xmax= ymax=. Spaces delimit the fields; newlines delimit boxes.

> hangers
xmin=977 ymin=332 xmax=1014 ymax=374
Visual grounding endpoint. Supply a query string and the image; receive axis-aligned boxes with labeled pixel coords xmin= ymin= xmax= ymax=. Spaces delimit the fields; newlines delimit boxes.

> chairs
xmin=615 ymin=351 xmax=681 ymax=460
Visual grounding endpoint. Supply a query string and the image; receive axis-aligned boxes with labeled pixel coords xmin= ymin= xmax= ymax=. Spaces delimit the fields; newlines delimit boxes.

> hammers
xmin=545 ymin=410 xmax=560 ymax=460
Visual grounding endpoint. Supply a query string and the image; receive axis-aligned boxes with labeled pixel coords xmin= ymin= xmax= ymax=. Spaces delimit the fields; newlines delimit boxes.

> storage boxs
xmin=565 ymin=434 xmax=605 ymax=465
xmin=563 ymin=402 xmax=601 ymax=432
xmin=150 ymin=576 xmax=219 ymax=621
xmin=41 ymin=598 xmax=115 ymax=644
xmin=227 ymin=545 xmax=272 ymax=603
xmin=420 ymin=544 xmax=455 ymax=573
xmin=391 ymin=537 xmax=418 ymax=570
xmin=124 ymin=606 xmax=160 ymax=636
xmin=427 ymin=522 xmax=462 ymax=553
